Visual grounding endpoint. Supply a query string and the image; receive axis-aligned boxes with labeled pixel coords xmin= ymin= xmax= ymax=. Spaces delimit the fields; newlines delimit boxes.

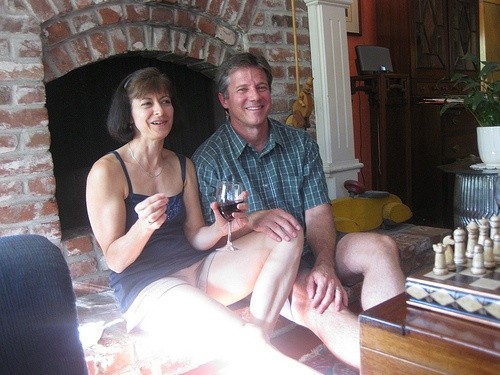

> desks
xmin=351 ymin=73 xmax=412 ymax=207
xmin=440 ymin=156 xmax=500 ymax=229
xmin=357 ymin=292 xmax=500 ymax=375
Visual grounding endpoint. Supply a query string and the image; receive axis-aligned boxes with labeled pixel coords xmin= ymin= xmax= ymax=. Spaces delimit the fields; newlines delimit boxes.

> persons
xmin=191 ymin=52 xmax=405 ymax=369
xmin=85 ymin=67 xmax=327 ymax=375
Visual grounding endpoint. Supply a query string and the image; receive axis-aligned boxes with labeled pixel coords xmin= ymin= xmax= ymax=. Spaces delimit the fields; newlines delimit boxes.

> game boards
xmin=403 ymin=250 xmax=500 ymax=329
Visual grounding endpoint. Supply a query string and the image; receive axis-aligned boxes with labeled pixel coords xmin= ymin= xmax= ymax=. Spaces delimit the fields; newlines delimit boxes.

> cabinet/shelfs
xmin=377 ymin=0 xmax=480 ymax=226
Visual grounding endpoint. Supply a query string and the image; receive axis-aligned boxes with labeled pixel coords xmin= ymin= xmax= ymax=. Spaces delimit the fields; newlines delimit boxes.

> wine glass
xmin=215 ymin=181 xmax=243 ymax=252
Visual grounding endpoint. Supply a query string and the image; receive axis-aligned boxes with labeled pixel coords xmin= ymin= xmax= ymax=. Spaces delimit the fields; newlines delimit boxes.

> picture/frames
xmin=345 ymin=0 xmax=362 ymax=36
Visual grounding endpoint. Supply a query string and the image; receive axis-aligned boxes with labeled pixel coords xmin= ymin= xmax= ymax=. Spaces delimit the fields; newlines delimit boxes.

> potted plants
xmin=436 ymin=53 xmax=500 ymax=167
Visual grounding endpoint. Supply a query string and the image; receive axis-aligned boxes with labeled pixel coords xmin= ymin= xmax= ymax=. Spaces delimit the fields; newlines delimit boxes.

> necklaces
xmin=127 ymin=142 xmax=163 ymax=177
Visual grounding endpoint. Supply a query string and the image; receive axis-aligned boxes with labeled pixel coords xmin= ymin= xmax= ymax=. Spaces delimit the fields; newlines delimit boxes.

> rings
xmin=146 ymin=217 xmax=154 ymax=224
